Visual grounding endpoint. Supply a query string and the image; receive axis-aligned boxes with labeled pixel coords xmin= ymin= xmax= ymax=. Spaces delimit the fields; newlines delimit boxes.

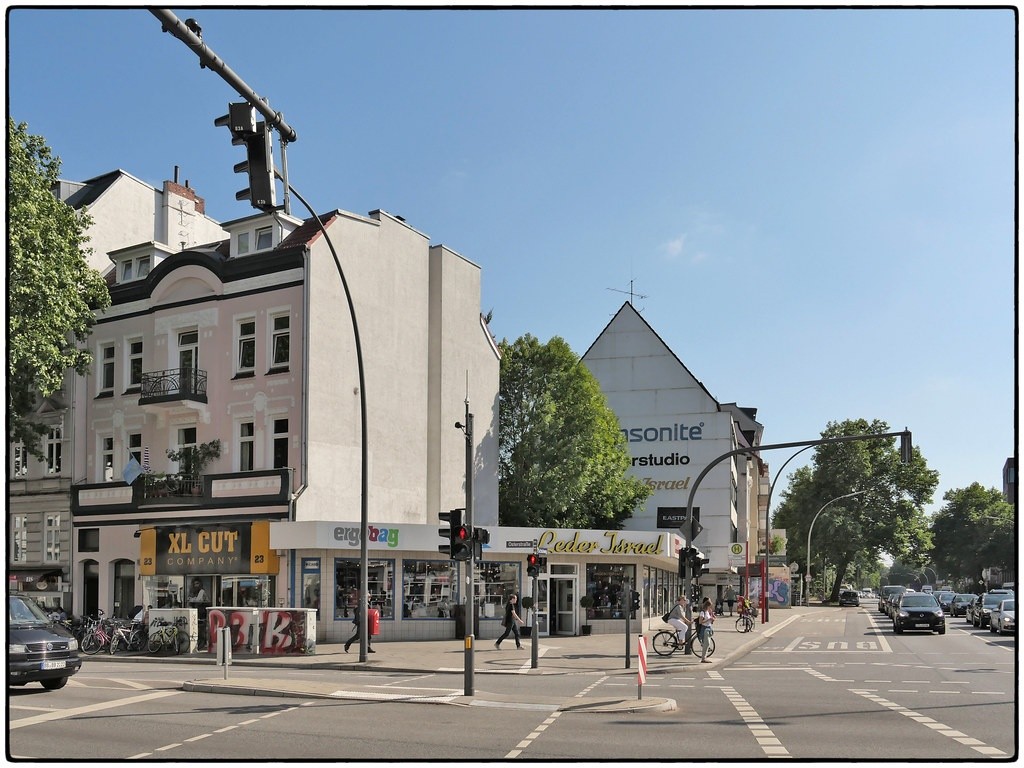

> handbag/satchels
xmin=501 ymin=614 xmax=507 ymax=627
xmin=662 ymin=612 xmax=670 ymax=623
xmin=694 ymin=610 xmax=705 ymax=634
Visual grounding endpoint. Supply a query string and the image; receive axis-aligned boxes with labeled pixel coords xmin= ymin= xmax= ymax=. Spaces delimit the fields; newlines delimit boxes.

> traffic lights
xmin=692 ymin=556 xmax=710 ymax=579
xmin=526 ymin=554 xmax=540 ymax=578
xmin=438 ymin=508 xmax=458 ymax=560
xmin=522 ymin=596 xmax=534 ymax=608
xmin=229 ymin=120 xmax=277 ymax=215
xmin=452 ymin=524 xmax=471 ymax=561
xmin=688 ymin=548 xmax=699 ymax=567
xmin=214 ymin=100 xmax=256 ymax=138
xmin=632 ymin=590 xmax=642 ymax=613
xmin=474 ymin=542 xmax=482 ymax=560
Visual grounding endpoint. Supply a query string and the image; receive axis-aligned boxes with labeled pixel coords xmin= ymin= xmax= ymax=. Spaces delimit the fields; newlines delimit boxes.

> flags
xmin=122 ymin=458 xmax=142 ymax=485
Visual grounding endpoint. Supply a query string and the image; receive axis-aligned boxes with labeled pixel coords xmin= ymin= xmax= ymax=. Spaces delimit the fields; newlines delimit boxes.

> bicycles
xmin=651 ymin=619 xmax=716 ymax=659
xmin=58 ymin=608 xmax=192 ymax=656
xmin=737 ymin=594 xmax=756 ymax=633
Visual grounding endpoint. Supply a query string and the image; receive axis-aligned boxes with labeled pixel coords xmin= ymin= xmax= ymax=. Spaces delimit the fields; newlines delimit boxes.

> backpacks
xmin=352 ymin=607 xmax=359 ymax=625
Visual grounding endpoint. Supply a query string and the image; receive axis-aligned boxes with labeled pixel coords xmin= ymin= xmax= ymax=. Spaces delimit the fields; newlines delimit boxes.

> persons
xmin=193 ymin=579 xmax=208 ymax=602
xmin=344 ymin=592 xmax=376 ymax=653
xmin=41 ymin=606 xmax=67 ymax=620
xmin=145 ymin=605 xmax=152 ymax=624
xmin=699 ymin=597 xmax=716 ymax=663
xmin=668 ymin=595 xmax=692 ymax=643
xmin=724 ymin=584 xmax=738 ymax=616
xmin=494 ymin=594 xmax=525 ymax=650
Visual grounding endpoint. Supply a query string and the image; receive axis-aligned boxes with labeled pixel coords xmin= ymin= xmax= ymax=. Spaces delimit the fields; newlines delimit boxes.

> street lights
xmin=803 ymin=490 xmax=865 ymax=607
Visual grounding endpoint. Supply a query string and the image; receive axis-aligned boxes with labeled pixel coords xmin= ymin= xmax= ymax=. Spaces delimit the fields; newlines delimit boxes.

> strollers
xmin=715 ymin=599 xmax=724 ymax=616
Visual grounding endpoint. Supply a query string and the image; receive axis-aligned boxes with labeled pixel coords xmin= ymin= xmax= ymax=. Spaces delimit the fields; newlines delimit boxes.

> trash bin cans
xmin=455 ymin=604 xmax=480 ymax=640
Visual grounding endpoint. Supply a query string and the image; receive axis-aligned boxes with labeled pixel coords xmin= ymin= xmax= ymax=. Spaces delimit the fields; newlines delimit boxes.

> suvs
xmin=990 ymin=589 xmax=1014 ymax=596
xmin=973 ymin=593 xmax=1015 ymax=629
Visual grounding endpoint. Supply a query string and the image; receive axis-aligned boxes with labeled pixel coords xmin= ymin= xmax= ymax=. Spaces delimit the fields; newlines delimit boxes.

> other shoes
xmin=494 ymin=643 xmax=500 ymax=650
xmin=368 ymin=647 xmax=376 ymax=653
xmin=517 ymin=647 xmax=525 ymax=650
xmin=344 ymin=644 xmax=349 ymax=654
xmin=701 ymin=660 xmax=713 ymax=663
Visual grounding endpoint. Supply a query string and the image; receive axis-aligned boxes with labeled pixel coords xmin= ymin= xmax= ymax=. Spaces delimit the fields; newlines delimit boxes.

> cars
xmin=839 ymin=590 xmax=861 ymax=607
xmin=878 ymin=584 xmax=953 ymax=619
xmin=950 ymin=593 xmax=980 ymax=618
xmin=938 ymin=593 xmax=959 ymax=612
xmin=9 ymin=595 xmax=82 ymax=693
xmin=892 ymin=592 xmax=947 ymax=634
xmin=966 ymin=597 xmax=977 ymax=624
xmin=991 ymin=599 xmax=1015 ymax=635
xmin=862 ymin=588 xmax=873 ymax=595
